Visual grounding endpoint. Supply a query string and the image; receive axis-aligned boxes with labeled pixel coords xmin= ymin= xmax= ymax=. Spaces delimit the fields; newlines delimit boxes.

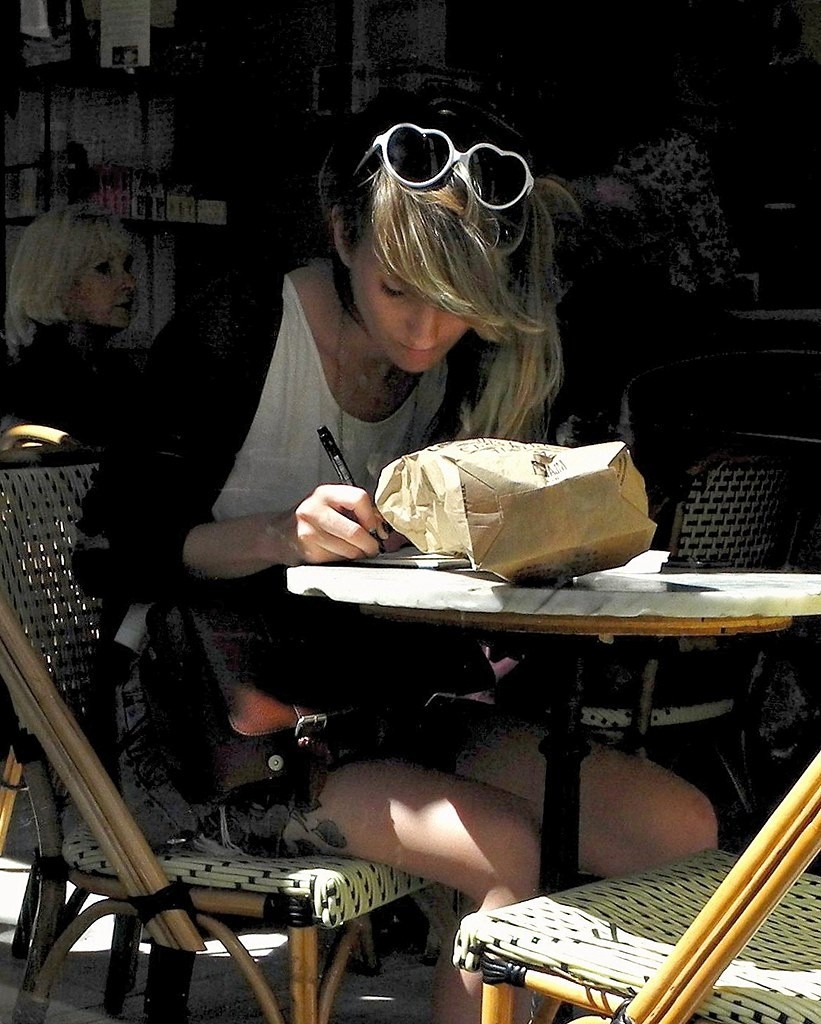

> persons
xmin=69 ymin=84 xmax=718 ymax=1024
xmin=0 ymin=202 xmax=158 ymax=459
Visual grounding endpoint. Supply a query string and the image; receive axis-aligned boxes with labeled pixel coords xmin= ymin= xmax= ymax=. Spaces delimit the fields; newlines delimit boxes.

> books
xmin=345 ymin=546 xmax=470 ymax=569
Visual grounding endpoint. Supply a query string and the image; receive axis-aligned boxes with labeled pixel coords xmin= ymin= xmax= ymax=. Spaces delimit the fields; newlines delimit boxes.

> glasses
xmin=353 ymin=122 xmax=535 ymax=210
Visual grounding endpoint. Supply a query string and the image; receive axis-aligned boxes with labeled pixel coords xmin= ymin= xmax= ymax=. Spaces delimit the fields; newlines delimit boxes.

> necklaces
xmin=337 ymin=300 xmax=422 ymax=497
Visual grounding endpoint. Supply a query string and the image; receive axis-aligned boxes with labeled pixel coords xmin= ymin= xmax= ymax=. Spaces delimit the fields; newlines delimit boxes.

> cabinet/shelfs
xmin=2 ymin=55 xmax=234 ymax=233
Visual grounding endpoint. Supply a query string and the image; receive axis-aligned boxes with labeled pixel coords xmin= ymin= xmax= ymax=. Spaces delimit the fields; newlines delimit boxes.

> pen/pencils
xmin=315 ymin=424 xmax=387 ymax=556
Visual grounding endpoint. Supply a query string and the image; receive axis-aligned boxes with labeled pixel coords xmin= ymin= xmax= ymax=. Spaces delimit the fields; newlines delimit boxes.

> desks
xmin=284 ymin=564 xmax=792 ymax=1024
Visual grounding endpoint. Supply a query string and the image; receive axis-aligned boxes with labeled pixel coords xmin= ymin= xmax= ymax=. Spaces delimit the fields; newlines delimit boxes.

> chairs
xmin=451 ymin=746 xmax=821 ymax=1024
xmin=582 ymin=457 xmax=807 ymax=817
xmin=1 ymin=441 xmax=469 ymax=1024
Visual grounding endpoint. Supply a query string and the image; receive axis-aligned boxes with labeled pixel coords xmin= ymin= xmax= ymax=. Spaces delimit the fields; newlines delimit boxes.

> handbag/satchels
xmin=139 ymin=588 xmax=397 ymax=799
